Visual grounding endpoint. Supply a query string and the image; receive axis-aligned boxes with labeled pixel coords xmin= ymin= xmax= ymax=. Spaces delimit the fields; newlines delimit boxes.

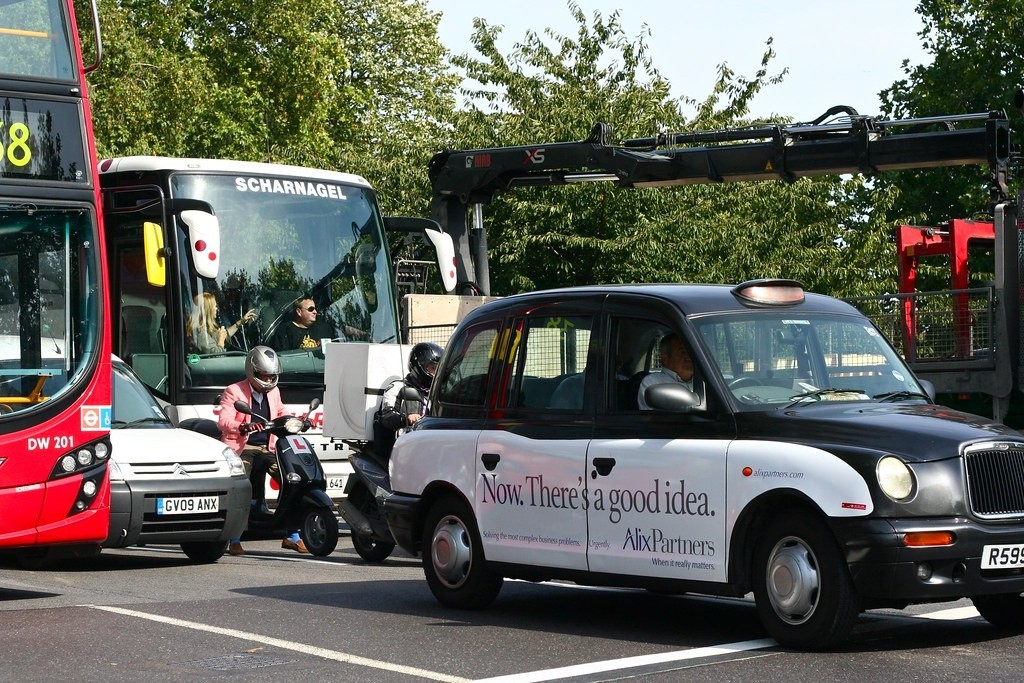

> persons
xmin=217 ymin=346 xmax=317 ymax=555
xmin=186 ymin=292 xmax=257 ymax=357
xmin=636 ymin=333 xmax=706 ymax=411
xmin=374 ymin=342 xmax=447 ymax=456
xmin=270 ymin=295 xmax=366 ymax=352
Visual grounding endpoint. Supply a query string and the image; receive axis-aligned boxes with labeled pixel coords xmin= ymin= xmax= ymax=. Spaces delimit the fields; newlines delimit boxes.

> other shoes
xmin=229 ymin=542 xmax=245 ymax=555
xmin=281 ymin=538 xmax=308 ymax=553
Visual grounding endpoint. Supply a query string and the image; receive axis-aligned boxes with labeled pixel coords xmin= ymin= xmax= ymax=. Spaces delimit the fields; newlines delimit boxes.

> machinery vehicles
xmin=425 ymin=105 xmax=1024 ymax=433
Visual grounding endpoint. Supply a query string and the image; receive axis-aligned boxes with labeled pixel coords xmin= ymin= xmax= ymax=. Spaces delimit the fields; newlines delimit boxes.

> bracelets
xmin=236 ymin=322 xmax=242 ymax=327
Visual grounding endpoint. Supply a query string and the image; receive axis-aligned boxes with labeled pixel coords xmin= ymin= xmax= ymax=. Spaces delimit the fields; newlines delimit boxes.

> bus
xmin=0 ymin=0 xmax=171 ymax=561
xmin=94 ymin=153 xmax=463 ymax=521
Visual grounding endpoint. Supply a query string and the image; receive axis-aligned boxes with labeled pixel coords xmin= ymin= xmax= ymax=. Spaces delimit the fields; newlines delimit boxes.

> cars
xmin=383 ymin=280 xmax=1024 ymax=653
xmin=0 ymin=334 xmax=254 ymax=563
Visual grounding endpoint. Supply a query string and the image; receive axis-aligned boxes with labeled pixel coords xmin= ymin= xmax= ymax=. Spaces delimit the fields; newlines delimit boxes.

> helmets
xmin=244 ymin=345 xmax=283 ymax=393
xmin=409 ymin=342 xmax=445 ymax=389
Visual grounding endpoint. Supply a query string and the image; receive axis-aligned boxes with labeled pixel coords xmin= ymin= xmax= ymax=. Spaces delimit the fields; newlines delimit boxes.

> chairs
xmin=261 ymin=289 xmax=303 ymax=350
xmin=120 ymin=306 xmax=164 ymax=358
xmin=616 ymin=370 xmax=650 ymax=411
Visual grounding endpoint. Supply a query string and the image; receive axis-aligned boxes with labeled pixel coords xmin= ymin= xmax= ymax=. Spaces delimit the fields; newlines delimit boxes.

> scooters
xmin=336 ymin=388 xmax=423 ymax=566
xmin=178 ymin=400 xmax=340 ymax=561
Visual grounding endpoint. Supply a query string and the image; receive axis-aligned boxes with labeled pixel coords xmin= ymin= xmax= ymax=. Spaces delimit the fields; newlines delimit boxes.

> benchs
xmin=445 ymin=371 xmax=584 ymax=411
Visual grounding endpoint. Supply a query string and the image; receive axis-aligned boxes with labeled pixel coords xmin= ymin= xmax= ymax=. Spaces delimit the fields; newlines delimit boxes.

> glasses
xmin=300 ymin=306 xmax=320 ymax=312
xmin=259 ymin=373 xmax=275 ymax=380
